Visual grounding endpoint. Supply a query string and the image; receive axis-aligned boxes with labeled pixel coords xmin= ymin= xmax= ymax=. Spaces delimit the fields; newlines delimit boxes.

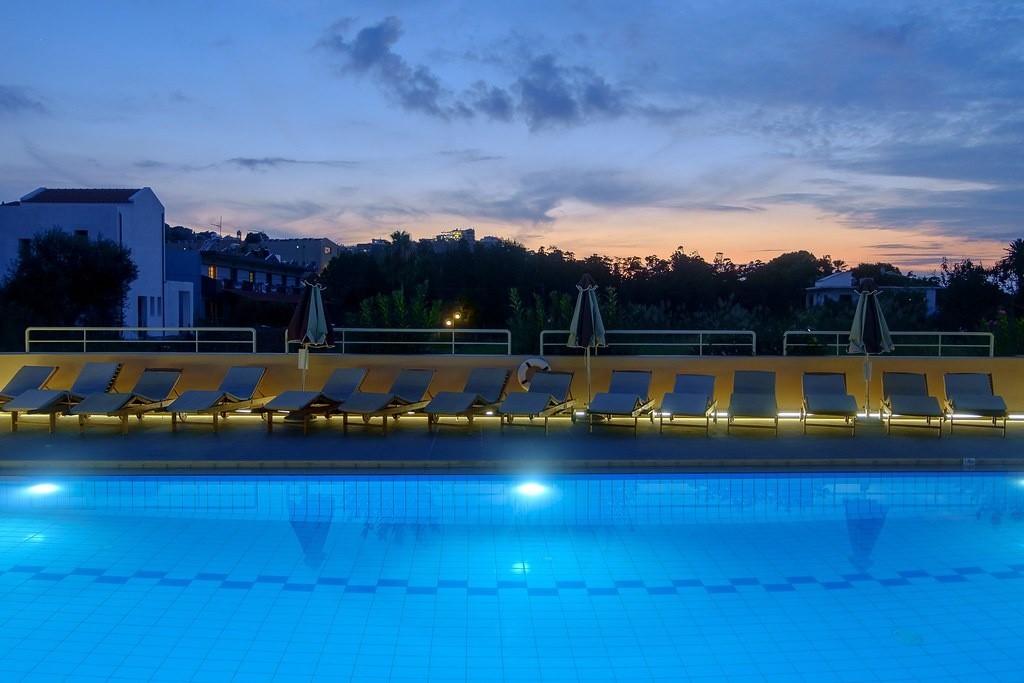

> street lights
xmin=446 ymin=311 xmax=461 ymax=354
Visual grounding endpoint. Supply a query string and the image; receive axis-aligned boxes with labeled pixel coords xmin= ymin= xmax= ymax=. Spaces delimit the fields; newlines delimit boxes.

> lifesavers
xmin=517 ymin=359 xmax=552 ymax=391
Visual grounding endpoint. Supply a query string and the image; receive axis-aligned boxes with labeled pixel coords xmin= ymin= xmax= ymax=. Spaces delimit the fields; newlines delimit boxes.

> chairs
xmin=799 ymin=370 xmax=859 ymax=436
xmin=335 ymin=367 xmax=438 ymax=435
xmin=725 ymin=369 xmax=779 ymax=439
xmin=162 ymin=364 xmax=277 ymax=433
xmin=260 ymin=368 xmax=369 ymax=435
xmin=494 ymin=370 xmax=579 ymax=438
xmin=0 ymin=365 xmax=59 ymax=414
xmin=879 ymin=370 xmax=944 ymax=438
xmin=942 ymin=372 xmax=1009 ymax=438
xmin=65 ymin=366 xmax=184 ymax=436
xmin=0 ymin=361 xmax=127 ymax=434
xmin=586 ymin=368 xmax=658 ymax=436
xmin=660 ymin=372 xmax=718 ymax=439
xmin=421 ymin=367 xmax=513 ymax=435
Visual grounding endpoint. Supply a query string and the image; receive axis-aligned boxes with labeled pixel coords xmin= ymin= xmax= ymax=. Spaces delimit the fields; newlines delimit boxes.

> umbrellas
xmin=567 ymin=273 xmax=607 ymax=411
xmin=286 ymin=261 xmax=337 ymax=390
xmin=845 ymin=278 xmax=895 ymax=417
